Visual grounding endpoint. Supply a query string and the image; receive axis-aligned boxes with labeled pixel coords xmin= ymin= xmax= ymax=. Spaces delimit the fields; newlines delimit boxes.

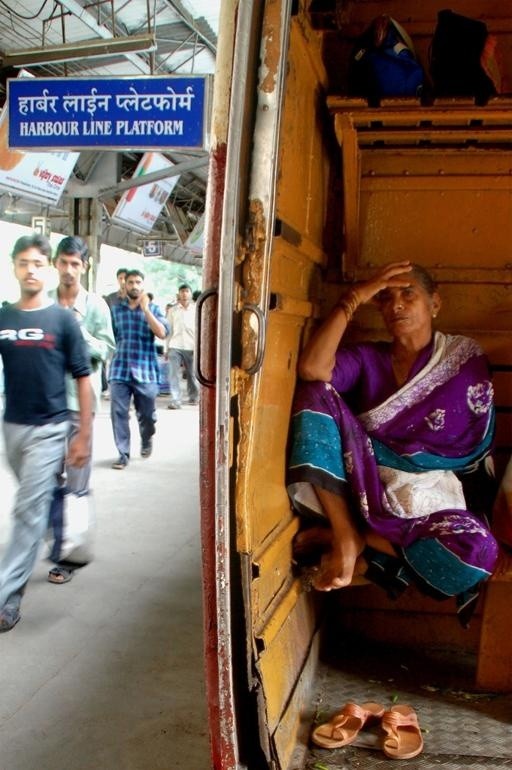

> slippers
xmin=311 ymin=702 xmax=384 ymax=749
xmin=381 ymin=704 xmax=423 ymax=759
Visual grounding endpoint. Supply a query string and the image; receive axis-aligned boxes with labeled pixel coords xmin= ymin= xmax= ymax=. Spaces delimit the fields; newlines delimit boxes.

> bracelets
xmin=333 ymin=285 xmax=362 ymax=323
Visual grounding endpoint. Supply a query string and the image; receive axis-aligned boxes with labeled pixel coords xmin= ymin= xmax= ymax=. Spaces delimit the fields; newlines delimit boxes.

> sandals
xmin=48 ymin=567 xmax=72 ymax=583
xmin=0 ymin=608 xmax=21 ymax=631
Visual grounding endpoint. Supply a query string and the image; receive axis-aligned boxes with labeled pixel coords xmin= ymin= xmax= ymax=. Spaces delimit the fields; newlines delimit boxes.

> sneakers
xmin=141 ymin=440 xmax=152 ymax=456
xmin=188 ymin=398 xmax=198 ymax=405
xmin=113 ymin=456 xmax=129 ymax=468
xmin=168 ymin=401 xmax=180 ymax=409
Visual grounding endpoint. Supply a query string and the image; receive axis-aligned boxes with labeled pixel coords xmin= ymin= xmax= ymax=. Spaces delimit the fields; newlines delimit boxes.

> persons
xmin=285 ymin=259 xmax=500 ymax=632
xmin=0 ymin=235 xmax=95 ymax=633
xmin=104 ymin=268 xmax=202 ymax=470
xmin=47 ymin=237 xmax=117 ymax=497
